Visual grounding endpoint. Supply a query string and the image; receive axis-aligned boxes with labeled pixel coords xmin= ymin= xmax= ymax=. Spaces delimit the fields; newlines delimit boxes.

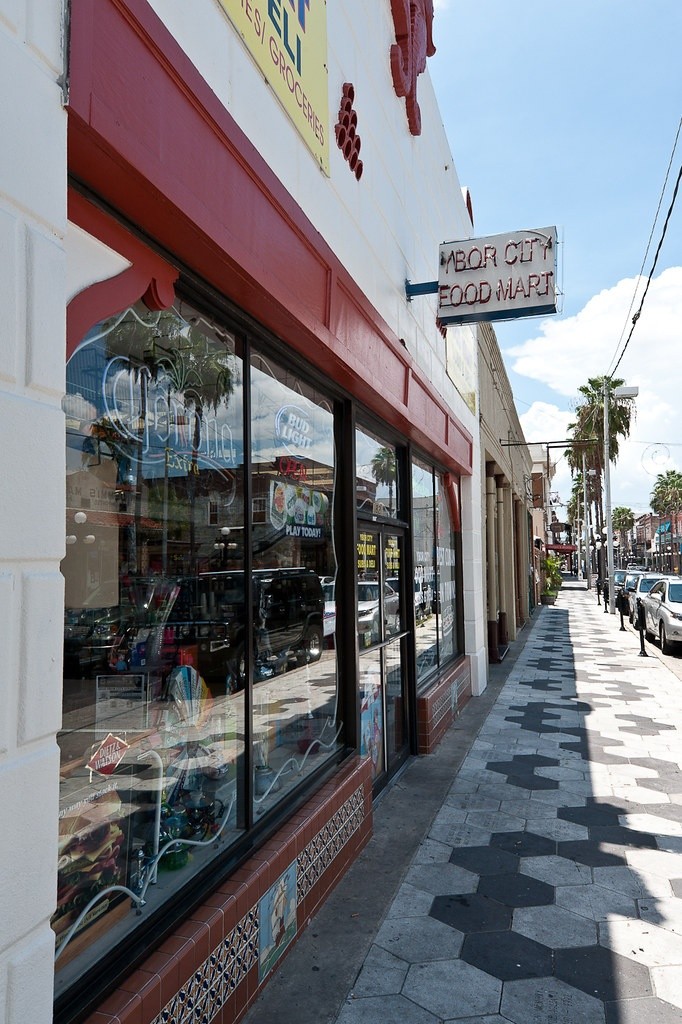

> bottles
xmin=185 ymin=792 xmax=209 ymax=836
xmin=162 ymin=806 xmax=188 ymax=870
xmin=130 ymin=849 xmax=147 ymax=908
xmin=154 ymin=773 xmax=171 ymax=847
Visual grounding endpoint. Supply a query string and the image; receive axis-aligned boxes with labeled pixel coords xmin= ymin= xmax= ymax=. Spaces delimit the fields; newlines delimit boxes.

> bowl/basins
xmin=297 ymin=739 xmax=320 ymax=752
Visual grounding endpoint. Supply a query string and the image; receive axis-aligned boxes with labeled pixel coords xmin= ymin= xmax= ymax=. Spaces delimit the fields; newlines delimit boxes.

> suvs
xmin=126 ymin=567 xmax=325 ymax=696
xmin=63 ymin=572 xmax=172 ymax=678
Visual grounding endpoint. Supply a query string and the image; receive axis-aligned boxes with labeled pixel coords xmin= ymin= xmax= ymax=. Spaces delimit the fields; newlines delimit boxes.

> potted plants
xmin=540 ymin=555 xmax=563 ymax=605
xmin=255 ymin=692 xmax=282 ymax=795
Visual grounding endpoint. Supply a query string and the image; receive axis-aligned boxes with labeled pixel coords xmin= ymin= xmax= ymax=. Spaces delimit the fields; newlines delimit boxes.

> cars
xmin=317 ymin=572 xmax=432 ymax=648
xmin=605 ymin=562 xmax=680 ymax=630
xmin=639 ymin=579 xmax=682 ymax=655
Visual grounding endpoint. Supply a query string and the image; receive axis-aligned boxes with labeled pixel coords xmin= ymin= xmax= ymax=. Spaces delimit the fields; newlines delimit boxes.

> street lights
xmin=594 ymin=526 xmax=619 ymax=582
xmin=214 ymin=526 xmax=238 ymax=571
xmin=600 ymin=374 xmax=640 ymax=614
xmin=581 ymin=453 xmax=597 ymax=591
